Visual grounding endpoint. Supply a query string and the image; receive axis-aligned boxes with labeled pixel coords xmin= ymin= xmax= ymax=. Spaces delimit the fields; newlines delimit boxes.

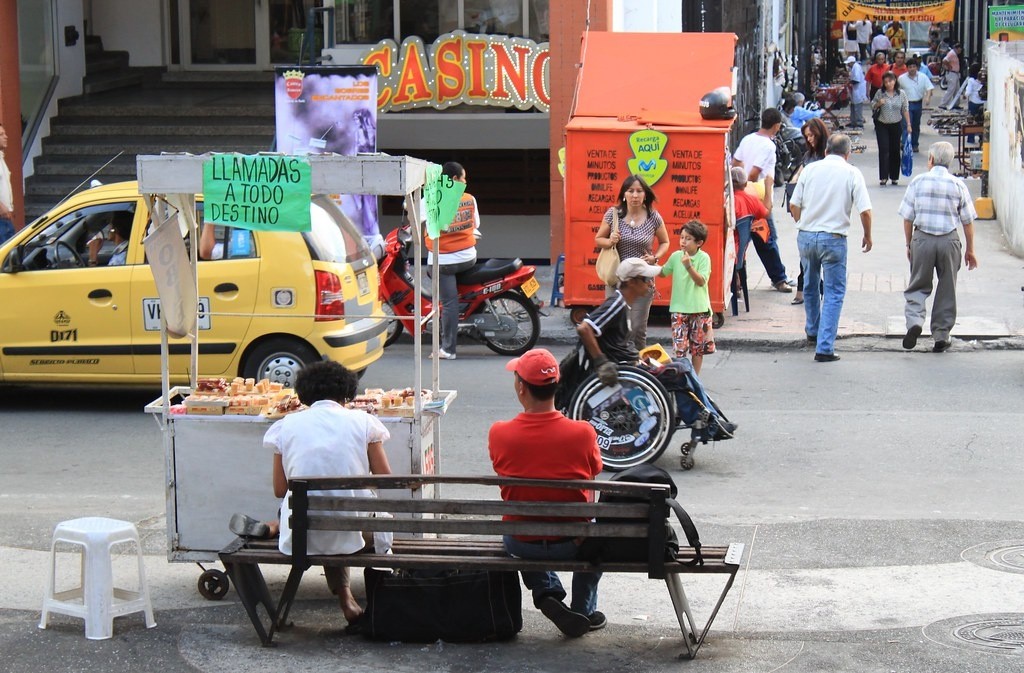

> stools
xmin=550 ymin=252 xmax=566 ymax=308
xmin=38 ymin=516 xmax=157 ymax=641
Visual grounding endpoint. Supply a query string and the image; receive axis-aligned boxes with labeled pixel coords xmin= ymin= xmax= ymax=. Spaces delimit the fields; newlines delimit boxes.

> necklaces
xmin=629 ymin=213 xmax=637 ymax=228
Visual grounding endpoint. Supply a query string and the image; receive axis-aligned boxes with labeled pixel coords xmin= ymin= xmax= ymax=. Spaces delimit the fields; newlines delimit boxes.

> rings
xmin=647 ymin=261 xmax=649 ymax=264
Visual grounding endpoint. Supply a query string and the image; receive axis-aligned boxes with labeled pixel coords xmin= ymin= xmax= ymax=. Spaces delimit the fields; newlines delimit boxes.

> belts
xmin=946 ymin=69 xmax=959 ymax=75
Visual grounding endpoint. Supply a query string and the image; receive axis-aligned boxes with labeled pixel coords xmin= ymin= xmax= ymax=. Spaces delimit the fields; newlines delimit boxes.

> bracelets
xmin=593 ymin=354 xmax=608 ymax=367
xmin=684 ymin=262 xmax=692 ymax=270
xmin=88 ymin=260 xmax=98 ymax=265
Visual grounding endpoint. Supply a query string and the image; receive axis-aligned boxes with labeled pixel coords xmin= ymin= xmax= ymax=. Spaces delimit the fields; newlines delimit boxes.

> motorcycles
xmin=370 ymin=210 xmax=551 ymax=357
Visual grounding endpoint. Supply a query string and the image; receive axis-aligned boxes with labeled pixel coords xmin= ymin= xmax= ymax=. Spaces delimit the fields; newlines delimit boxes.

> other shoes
xmin=770 ymin=280 xmax=793 ymax=293
xmin=901 ymin=145 xmax=905 ymax=150
xmin=933 ymin=337 xmax=953 ymax=352
xmin=814 ymin=352 xmax=841 ymax=362
xmin=736 ymin=287 xmax=743 ymax=298
xmin=953 ymin=106 xmax=964 ymax=110
xmin=807 ymin=332 xmax=817 ymax=341
xmin=913 ymin=145 xmax=921 ymax=154
xmin=691 ymin=421 xmax=737 ymax=441
xmin=846 ymin=123 xmax=864 ymax=128
xmin=538 ymin=598 xmax=590 ymax=637
xmin=344 ymin=612 xmax=363 ymax=634
xmin=902 ymin=325 xmax=922 ymax=350
xmin=583 ymin=611 xmax=608 ymax=629
xmin=938 ymin=105 xmax=948 ymax=110
xmin=229 ymin=513 xmax=271 ymax=538
xmin=428 ymin=349 xmax=457 ymax=360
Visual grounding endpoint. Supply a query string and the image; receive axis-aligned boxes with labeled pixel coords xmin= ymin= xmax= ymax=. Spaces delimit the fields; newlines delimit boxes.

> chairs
xmin=730 ymin=214 xmax=755 ymax=315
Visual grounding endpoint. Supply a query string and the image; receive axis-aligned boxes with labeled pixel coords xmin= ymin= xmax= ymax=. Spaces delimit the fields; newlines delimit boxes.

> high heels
xmin=820 ymin=291 xmax=824 ymax=301
xmin=791 ymin=290 xmax=804 ymax=304
xmin=880 ymin=177 xmax=888 ymax=186
xmin=892 ymin=180 xmax=898 ymax=185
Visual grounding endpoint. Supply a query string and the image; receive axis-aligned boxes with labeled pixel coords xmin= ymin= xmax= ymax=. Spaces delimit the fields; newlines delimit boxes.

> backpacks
xmin=591 ymin=465 xmax=706 ymax=567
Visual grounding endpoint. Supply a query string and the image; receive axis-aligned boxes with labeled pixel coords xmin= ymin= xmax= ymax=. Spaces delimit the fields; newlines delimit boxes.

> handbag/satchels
xmin=596 ymin=205 xmax=621 ymax=287
xmin=901 ymin=134 xmax=916 ymax=177
xmin=786 ymin=164 xmax=805 ymax=212
xmin=363 ymin=547 xmax=526 ymax=645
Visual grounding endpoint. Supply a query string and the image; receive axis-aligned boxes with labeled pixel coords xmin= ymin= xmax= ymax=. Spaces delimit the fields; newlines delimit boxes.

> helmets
xmin=699 ymin=91 xmax=737 ymax=120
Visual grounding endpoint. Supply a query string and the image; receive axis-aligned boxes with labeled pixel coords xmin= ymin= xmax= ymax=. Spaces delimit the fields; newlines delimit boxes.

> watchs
xmin=653 ymin=254 xmax=659 ymax=264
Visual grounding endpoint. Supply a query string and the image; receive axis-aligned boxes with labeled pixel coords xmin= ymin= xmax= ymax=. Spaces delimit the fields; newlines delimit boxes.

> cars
xmin=0 ymin=176 xmax=390 ymax=416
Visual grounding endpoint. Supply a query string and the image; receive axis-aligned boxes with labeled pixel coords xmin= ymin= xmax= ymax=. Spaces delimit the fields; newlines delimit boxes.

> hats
xmin=616 ymin=257 xmax=663 ymax=280
xmin=844 ymin=56 xmax=856 ymax=63
xmin=506 ymin=348 xmax=561 ymax=385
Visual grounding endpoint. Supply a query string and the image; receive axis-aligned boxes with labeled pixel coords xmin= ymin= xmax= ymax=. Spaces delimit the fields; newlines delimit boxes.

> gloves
xmin=592 ymin=354 xmax=620 ymax=388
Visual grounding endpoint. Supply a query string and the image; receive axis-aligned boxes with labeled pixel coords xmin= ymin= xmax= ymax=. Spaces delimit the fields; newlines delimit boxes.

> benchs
xmin=217 ymin=474 xmax=745 ymax=660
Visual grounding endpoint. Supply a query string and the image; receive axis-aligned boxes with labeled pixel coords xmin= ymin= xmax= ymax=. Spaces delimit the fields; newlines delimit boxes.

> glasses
xmin=626 ymin=275 xmax=655 ymax=283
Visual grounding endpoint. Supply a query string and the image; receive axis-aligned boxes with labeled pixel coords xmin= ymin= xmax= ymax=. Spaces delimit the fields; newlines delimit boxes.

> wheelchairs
xmin=554 ymin=334 xmax=736 ymax=474
xmin=772 ymin=124 xmax=808 ymax=187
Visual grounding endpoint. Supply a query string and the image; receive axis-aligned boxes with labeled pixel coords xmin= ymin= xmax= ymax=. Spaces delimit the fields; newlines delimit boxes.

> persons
xmin=732 ymin=108 xmax=796 ymax=292
xmin=897 ymin=141 xmax=978 ymax=352
xmin=731 ymin=166 xmax=774 ymax=298
xmin=88 ymin=211 xmax=135 ymax=267
xmin=645 ymin=220 xmax=717 ymax=378
xmin=790 ymin=133 xmax=873 ymax=361
xmin=264 ymin=360 xmax=391 ymax=623
xmin=198 ymin=222 xmax=255 ymax=261
xmin=0 ymin=123 xmax=15 ymax=269
xmin=595 ymin=174 xmax=670 ymax=350
xmin=489 ymin=349 xmax=607 ymax=637
xmin=576 ymin=257 xmax=738 ymax=442
xmin=781 ymin=16 xmax=987 ymax=186
xmin=406 ymin=163 xmax=480 ymax=360
xmin=792 ymin=118 xmax=829 ymax=305
